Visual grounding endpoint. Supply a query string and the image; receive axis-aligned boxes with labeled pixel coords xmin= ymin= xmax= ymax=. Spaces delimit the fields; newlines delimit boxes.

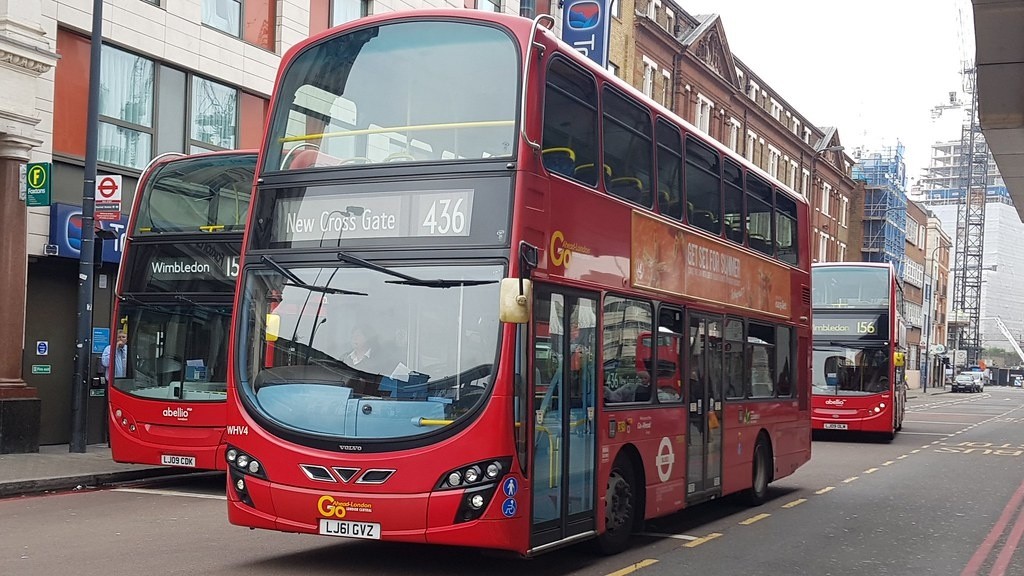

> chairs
xmin=713 ymin=219 xmax=731 ymax=239
xmin=765 ymin=240 xmax=781 ymax=257
xmin=731 ymin=226 xmax=751 ymax=248
xmin=574 ymin=163 xmax=611 ymax=189
xmin=670 ymin=201 xmax=693 ymax=224
xmin=748 ymin=233 xmax=766 ymax=253
xmin=542 ymin=146 xmax=575 ymax=176
xmin=642 ymin=190 xmax=670 ymax=215
xmin=694 ymin=211 xmax=713 ymax=232
xmin=611 ymin=178 xmax=643 ymax=204
xmin=382 ymin=151 xmax=418 ymax=165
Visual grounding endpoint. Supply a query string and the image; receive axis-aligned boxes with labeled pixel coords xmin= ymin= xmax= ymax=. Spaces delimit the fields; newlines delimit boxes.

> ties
xmin=120 ymin=349 xmax=127 ymax=377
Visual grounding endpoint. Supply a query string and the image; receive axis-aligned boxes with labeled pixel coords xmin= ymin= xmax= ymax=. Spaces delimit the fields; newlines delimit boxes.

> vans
xmin=960 ymin=371 xmax=985 ymax=393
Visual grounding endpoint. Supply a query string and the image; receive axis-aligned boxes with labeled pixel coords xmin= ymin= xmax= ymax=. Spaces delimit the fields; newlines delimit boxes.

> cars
xmin=951 ymin=374 xmax=977 ymax=393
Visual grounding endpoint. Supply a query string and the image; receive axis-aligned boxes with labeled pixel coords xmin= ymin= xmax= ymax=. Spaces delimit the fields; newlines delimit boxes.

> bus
xmin=92 ymin=142 xmax=355 ymax=472
xmin=225 ymin=9 xmax=816 ymax=562
xmin=811 ymin=260 xmax=907 ymax=440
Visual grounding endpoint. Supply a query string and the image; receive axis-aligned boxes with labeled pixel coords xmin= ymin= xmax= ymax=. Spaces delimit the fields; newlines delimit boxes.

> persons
xmin=101 ymin=330 xmax=127 ymax=448
xmin=336 ymin=323 xmax=390 ymax=374
